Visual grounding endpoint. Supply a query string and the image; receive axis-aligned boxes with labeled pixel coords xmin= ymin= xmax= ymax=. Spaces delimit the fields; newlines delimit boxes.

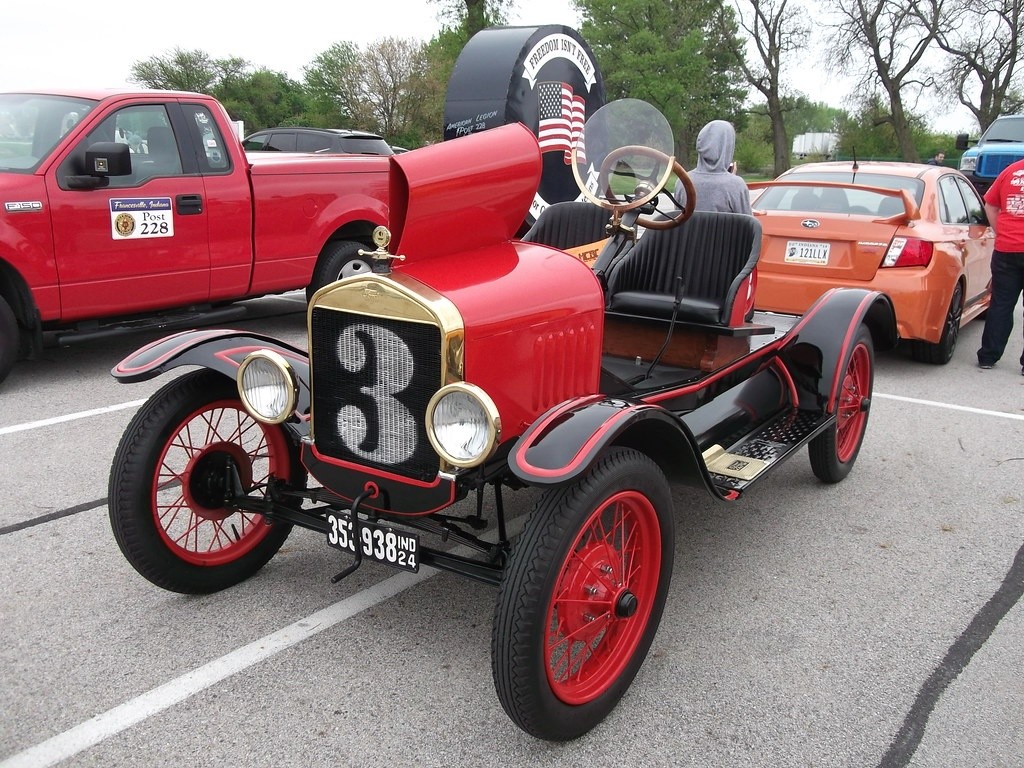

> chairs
xmin=521 ymin=201 xmax=638 ymax=286
xmin=792 ymin=188 xmax=905 ymax=216
xmin=608 ymin=211 xmax=765 ymax=336
xmin=134 ymin=127 xmax=173 ymax=175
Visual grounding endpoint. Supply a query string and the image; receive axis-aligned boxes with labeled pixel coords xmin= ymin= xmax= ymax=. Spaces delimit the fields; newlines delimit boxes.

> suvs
xmin=956 ymin=114 xmax=1024 ymax=204
xmin=239 ymin=128 xmax=395 ymax=156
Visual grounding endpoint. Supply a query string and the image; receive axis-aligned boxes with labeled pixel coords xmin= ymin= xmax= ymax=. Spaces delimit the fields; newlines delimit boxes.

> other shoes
xmin=979 ymin=362 xmax=992 ymax=369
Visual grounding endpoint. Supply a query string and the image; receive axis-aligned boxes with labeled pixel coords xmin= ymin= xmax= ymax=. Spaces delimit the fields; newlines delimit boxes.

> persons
xmin=975 ymin=159 xmax=1024 ymax=375
xmin=927 ymin=151 xmax=946 ymax=165
xmin=673 ymin=119 xmax=753 ymax=217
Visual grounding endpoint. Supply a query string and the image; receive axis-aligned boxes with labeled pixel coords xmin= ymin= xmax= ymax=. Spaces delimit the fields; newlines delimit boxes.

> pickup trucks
xmin=0 ymin=89 xmax=411 ymax=382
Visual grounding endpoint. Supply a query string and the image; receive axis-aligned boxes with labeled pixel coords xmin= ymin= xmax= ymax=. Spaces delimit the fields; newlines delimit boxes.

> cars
xmin=105 ymin=97 xmax=899 ymax=744
xmin=747 ymin=160 xmax=996 ymax=366
